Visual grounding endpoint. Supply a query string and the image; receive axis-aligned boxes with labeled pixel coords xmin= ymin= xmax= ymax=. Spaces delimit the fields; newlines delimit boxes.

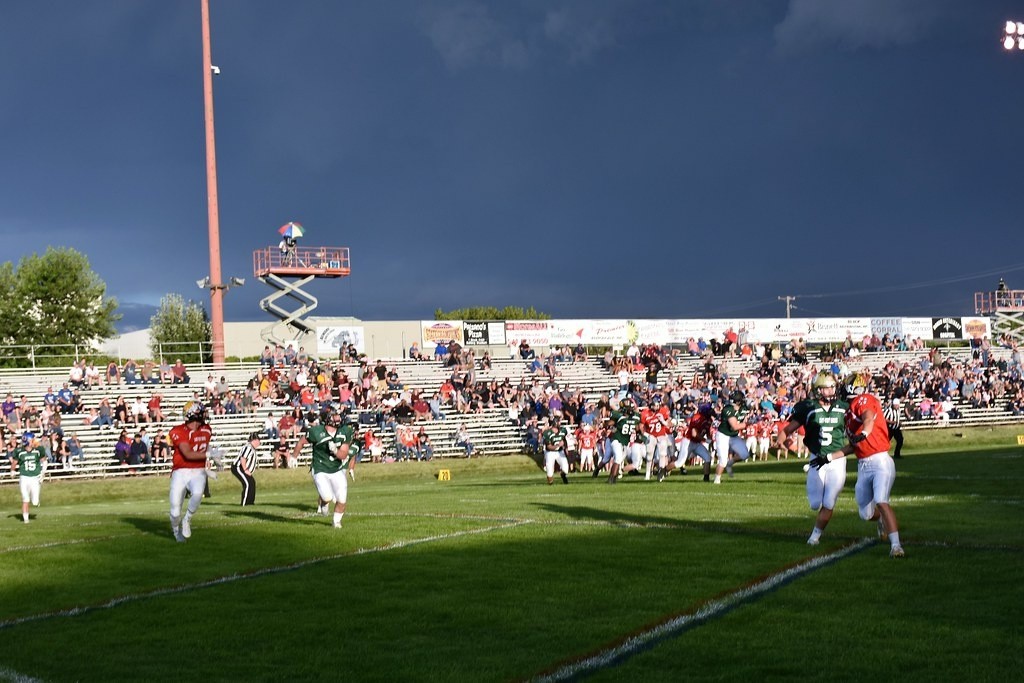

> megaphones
xmin=230 ymin=277 xmax=245 ymax=286
xmin=196 ymin=276 xmax=209 ymax=288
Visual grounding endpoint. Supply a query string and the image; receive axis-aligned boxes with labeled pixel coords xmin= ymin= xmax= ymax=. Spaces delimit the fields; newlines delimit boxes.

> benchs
xmin=0 ymin=348 xmax=1024 ymax=484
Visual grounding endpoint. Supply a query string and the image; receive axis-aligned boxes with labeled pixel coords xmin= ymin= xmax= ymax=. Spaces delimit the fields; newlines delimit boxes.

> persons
xmin=430 ymin=327 xmax=819 ymax=463
xmin=289 ymin=407 xmax=353 ymax=529
xmin=543 ymin=417 xmax=569 ymax=484
xmin=456 ymin=424 xmax=473 ymax=458
xmin=279 ymin=237 xmax=292 ymax=267
xmin=713 ymin=391 xmax=753 ymax=484
xmin=231 ymin=432 xmax=261 ymax=506
xmin=639 ymin=398 xmax=672 ymax=482
xmin=10 ymin=432 xmax=47 ymax=524
xmin=810 ymin=374 xmax=905 ymax=557
xmin=770 ymin=370 xmax=847 ymax=545
xmin=194 ymin=341 xmax=432 ymax=470
xmin=169 ymin=401 xmax=212 ymax=543
xmin=606 ymin=399 xmax=641 ymax=484
xmin=656 ymin=404 xmax=714 ymax=482
xmin=0 ymin=358 xmax=190 ymax=476
xmin=578 ymin=426 xmax=597 ymax=472
xmin=820 ymin=332 xmax=1024 ymax=422
xmin=883 ymin=398 xmax=904 ymax=459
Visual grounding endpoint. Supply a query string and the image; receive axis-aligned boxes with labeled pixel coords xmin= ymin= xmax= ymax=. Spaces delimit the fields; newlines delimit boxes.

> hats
xmin=892 ymin=398 xmax=899 ymax=404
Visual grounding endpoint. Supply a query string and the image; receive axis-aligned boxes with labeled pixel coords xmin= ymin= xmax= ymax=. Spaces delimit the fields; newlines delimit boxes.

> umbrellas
xmin=278 ymin=221 xmax=305 ymax=238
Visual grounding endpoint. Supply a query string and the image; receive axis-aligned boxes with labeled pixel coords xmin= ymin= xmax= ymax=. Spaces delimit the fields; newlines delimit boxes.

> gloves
xmin=810 ymin=453 xmax=829 ymax=471
xmin=329 ymin=441 xmax=337 ymax=456
xmin=288 ymin=455 xmax=297 ymax=469
xmin=849 ymin=432 xmax=866 ymax=447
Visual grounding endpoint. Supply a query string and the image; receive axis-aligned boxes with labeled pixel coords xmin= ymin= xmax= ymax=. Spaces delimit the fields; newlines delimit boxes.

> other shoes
xmin=657 ymin=467 xmax=665 ymax=483
xmin=561 ymin=472 xmax=569 ymax=484
xmin=174 ymin=528 xmax=186 ymax=543
xmin=333 ymin=522 xmax=341 ymax=529
xmin=714 ymin=474 xmax=721 ymax=484
xmin=807 ymin=536 xmax=819 ymax=546
xmin=321 ymin=504 xmax=328 ymax=517
xmin=726 ymin=466 xmax=734 ymax=478
xmin=181 ymin=519 xmax=192 ymax=539
xmin=889 ymin=545 xmax=904 ymax=557
xmin=877 ymin=518 xmax=888 ymax=542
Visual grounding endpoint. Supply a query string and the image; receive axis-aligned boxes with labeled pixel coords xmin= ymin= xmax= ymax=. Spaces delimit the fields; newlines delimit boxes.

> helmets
xmin=548 ymin=416 xmax=561 ymax=427
xmin=649 ymin=396 xmax=662 ymax=411
xmin=319 ymin=402 xmax=346 ymax=428
xmin=183 ymin=401 xmax=207 ymax=424
xmin=619 ymin=398 xmax=634 ymax=415
xmin=22 ymin=432 xmax=35 ymax=447
xmin=811 ymin=373 xmax=836 ymax=391
xmin=698 ymin=403 xmax=713 ymax=418
xmin=728 ymin=390 xmax=746 ymax=408
xmin=842 ymin=372 xmax=868 ymax=401
